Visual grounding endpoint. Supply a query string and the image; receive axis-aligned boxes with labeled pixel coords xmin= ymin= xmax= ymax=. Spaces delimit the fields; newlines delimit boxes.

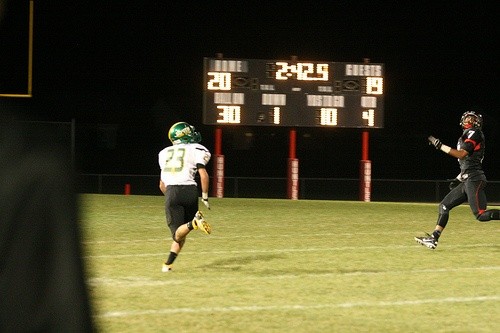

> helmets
xmin=168 ymin=121 xmax=202 ymax=145
xmin=460 ymin=111 xmax=483 ymax=128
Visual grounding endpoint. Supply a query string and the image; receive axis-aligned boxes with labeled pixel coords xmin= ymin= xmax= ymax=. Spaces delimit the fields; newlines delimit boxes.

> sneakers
xmin=162 ymin=263 xmax=173 ymax=273
xmin=415 ymin=235 xmax=437 ymax=249
xmin=192 ymin=210 xmax=212 ymax=236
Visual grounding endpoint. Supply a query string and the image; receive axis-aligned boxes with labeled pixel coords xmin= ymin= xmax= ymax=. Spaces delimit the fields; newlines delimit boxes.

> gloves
xmin=429 ymin=136 xmax=442 ymax=150
xmin=201 ymin=198 xmax=211 ymax=209
xmin=448 ymin=178 xmax=461 ymax=189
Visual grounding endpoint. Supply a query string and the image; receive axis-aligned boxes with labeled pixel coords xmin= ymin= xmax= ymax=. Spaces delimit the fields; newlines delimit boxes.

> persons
xmin=158 ymin=122 xmax=212 ymax=271
xmin=414 ymin=110 xmax=500 ymax=248
